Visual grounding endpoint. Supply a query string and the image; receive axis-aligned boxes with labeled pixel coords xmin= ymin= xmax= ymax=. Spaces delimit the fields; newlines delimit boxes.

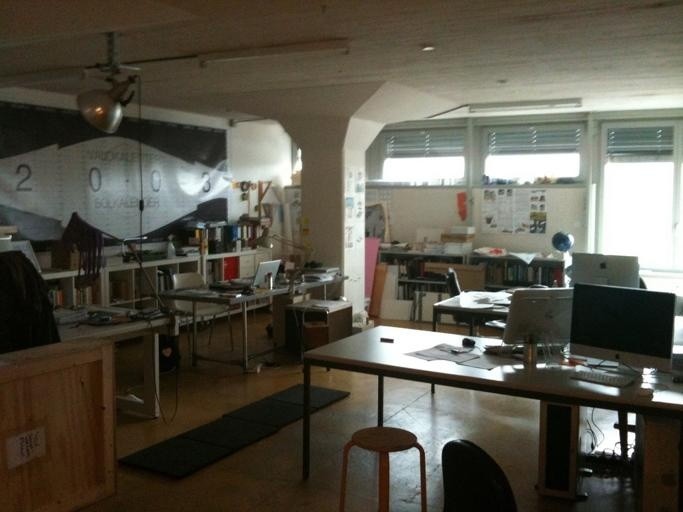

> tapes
xmin=240 ymin=182 xmax=250 ymax=192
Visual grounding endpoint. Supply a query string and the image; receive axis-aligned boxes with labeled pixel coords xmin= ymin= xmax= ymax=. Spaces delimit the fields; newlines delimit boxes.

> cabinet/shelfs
xmin=39 ymin=246 xmax=272 ymax=310
xmin=372 ymin=250 xmax=565 ymax=327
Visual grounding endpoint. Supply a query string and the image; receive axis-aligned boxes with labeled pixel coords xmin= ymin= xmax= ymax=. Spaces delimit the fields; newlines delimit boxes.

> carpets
xmin=118 ymin=383 xmax=350 ymax=481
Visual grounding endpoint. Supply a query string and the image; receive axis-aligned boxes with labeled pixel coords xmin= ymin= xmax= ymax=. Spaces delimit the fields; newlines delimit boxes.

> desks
xmin=301 ymin=323 xmax=683 ymax=512
xmin=161 ymin=271 xmax=349 ymax=371
xmin=52 ymin=294 xmax=233 ymax=418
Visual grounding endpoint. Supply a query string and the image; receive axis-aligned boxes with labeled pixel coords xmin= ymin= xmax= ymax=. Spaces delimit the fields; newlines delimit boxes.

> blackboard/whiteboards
xmin=282 ymin=184 xmax=594 ymax=328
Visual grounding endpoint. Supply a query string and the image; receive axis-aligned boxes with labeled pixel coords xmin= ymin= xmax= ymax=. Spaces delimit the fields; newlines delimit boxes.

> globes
xmin=552 ymin=231 xmax=574 ymax=257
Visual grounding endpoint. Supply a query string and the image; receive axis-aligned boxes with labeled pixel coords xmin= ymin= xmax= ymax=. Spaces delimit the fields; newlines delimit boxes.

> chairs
xmin=441 ymin=439 xmax=518 ymax=512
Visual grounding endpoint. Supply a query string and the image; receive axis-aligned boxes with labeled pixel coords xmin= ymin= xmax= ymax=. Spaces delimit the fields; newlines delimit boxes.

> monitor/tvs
xmin=571 ymin=251 xmax=641 ymax=288
xmin=503 ymin=287 xmax=575 ymax=376
xmin=569 ymin=281 xmax=677 ymax=378
xmin=252 ymin=259 xmax=282 ymax=290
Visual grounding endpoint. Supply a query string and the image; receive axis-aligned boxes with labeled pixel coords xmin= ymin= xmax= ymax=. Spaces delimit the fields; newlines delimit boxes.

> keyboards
xmin=484 ymin=342 xmax=564 ymax=356
xmin=570 ymin=367 xmax=635 ymax=389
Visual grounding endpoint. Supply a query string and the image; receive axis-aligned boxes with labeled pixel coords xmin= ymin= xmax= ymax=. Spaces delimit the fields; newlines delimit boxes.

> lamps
xmin=77 ymin=30 xmax=140 ymax=134
xmin=468 ymin=97 xmax=583 ymax=113
xmin=197 ymin=37 xmax=350 ymax=68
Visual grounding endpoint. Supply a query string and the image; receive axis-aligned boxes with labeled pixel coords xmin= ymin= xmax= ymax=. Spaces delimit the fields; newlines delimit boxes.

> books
xmin=157 ymin=267 xmax=176 ymax=291
xmin=49 ymin=285 xmax=94 ymax=307
xmin=485 ymin=262 xmax=562 ymax=288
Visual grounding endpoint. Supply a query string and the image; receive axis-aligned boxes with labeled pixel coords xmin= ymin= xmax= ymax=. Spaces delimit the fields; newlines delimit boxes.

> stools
xmin=339 ymin=427 xmax=426 ymax=512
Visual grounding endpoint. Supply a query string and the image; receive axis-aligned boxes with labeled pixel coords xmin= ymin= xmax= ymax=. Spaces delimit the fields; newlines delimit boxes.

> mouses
xmin=462 ymin=335 xmax=475 ymax=348
xmin=638 ymin=386 xmax=654 ymax=397
